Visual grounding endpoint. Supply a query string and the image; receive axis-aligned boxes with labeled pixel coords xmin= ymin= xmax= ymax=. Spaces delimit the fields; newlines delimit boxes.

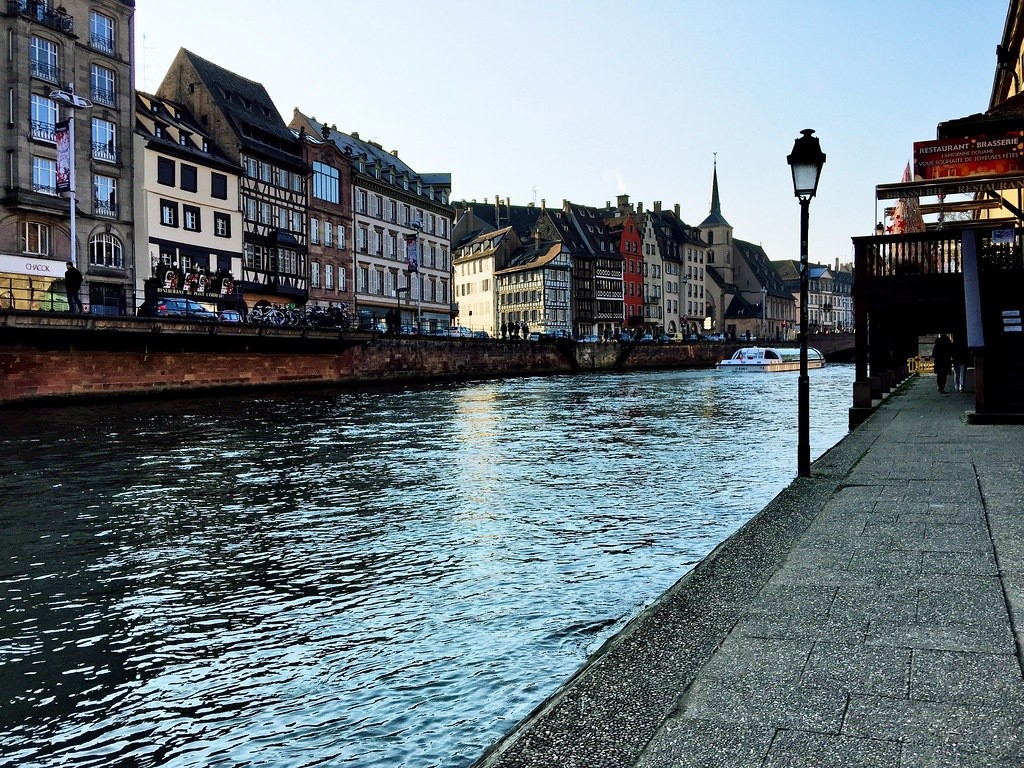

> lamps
xmin=874 ymin=222 xmax=884 ymax=235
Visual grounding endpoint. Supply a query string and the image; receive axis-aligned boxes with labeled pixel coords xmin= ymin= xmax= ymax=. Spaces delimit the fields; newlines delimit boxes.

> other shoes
xmin=955 ymin=389 xmax=959 ymax=393
xmin=937 ymin=387 xmax=944 ymax=392
xmin=959 ymin=388 xmax=963 ymax=392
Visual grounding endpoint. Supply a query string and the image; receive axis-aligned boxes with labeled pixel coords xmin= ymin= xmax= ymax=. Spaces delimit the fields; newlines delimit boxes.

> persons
xmin=604 ymin=327 xmax=614 ymax=343
xmin=385 ymin=309 xmax=402 ymax=335
xmin=64 ymin=262 xmax=85 ymax=313
xmin=500 ymin=320 xmax=529 ymax=341
xmin=932 ymin=332 xmax=968 ymax=393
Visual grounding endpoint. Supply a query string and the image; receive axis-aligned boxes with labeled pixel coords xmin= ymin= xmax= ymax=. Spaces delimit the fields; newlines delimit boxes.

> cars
xmin=598 ymin=335 xmax=618 ymax=343
xmin=640 ymin=334 xmax=653 ymax=341
xmin=138 ymin=297 xmax=216 ymax=318
xmin=437 ymin=326 xmax=489 ymax=338
xmin=657 ymin=334 xmax=669 ymax=344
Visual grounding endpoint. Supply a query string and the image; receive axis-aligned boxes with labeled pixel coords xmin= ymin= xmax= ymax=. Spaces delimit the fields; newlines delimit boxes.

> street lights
xmin=396 ymin=288 xmax=410 ymax=339
xmin=786 ymin=128 xmax=826 ymax=476
xmin=49 ymin=90 xmax=93 ymax=269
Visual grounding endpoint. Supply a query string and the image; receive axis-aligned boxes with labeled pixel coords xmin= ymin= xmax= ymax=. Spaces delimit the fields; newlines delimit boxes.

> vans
xmin=667 ymin=333 xmax=683 ymax=342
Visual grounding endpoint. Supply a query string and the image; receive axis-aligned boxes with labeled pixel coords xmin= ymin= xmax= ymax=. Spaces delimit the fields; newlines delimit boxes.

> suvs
xmin=577 ymin=335 xmax=599 ymax=343
xmin=706 ymin=332 xmax=724 ymax=342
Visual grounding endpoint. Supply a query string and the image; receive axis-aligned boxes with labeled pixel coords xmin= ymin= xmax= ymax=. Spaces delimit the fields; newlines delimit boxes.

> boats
xmin=715 ymin=345 xmax=826 ymax=372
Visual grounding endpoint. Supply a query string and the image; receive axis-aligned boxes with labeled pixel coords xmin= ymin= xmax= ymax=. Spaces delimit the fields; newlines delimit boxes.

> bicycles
xmin=247 ymin=303 xmax=301 ymax=327
